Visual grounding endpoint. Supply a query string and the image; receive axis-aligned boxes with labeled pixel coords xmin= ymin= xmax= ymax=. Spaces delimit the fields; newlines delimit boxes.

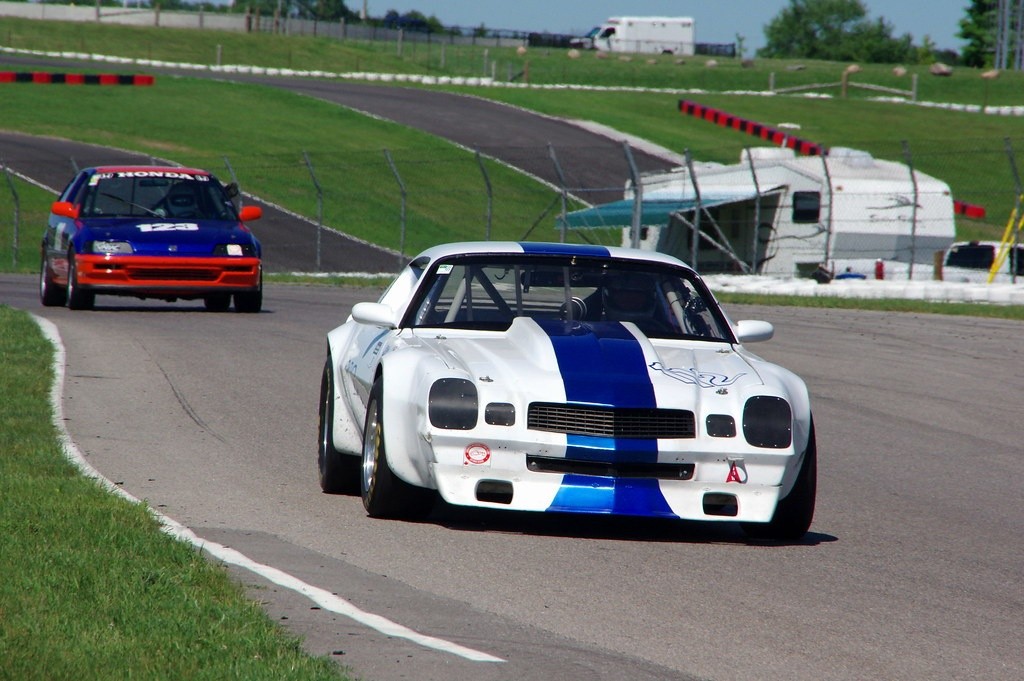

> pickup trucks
xmin=826 ymin=240 xmax=1024 ymax=287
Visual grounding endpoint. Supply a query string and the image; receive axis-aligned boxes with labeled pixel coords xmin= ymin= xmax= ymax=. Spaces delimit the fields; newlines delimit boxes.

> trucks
xmin=569 ymin=16 xmax=696 ymax=56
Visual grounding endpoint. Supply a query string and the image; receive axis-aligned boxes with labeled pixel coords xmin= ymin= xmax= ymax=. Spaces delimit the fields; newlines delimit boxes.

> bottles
xmin=875 ymin=258 xmax=884 ymax=279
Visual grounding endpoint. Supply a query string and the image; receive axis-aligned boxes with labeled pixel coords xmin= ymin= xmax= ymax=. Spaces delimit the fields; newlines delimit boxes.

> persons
xmin=163 ymin=178 xmax=198 ymax=215
xmin=597 ymin=269 xmax=650 ymax=320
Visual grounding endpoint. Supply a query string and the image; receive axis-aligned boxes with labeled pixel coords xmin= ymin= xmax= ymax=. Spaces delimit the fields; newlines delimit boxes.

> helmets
xmin=601 ymin=264 xmax=657 ymax=322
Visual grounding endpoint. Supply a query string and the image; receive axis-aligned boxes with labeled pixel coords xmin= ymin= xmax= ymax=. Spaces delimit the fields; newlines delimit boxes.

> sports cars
xmin=40 ymin=165 xmax=264 ymax=313
xmin=319 ymin=240 xmax=816 ymax=547
